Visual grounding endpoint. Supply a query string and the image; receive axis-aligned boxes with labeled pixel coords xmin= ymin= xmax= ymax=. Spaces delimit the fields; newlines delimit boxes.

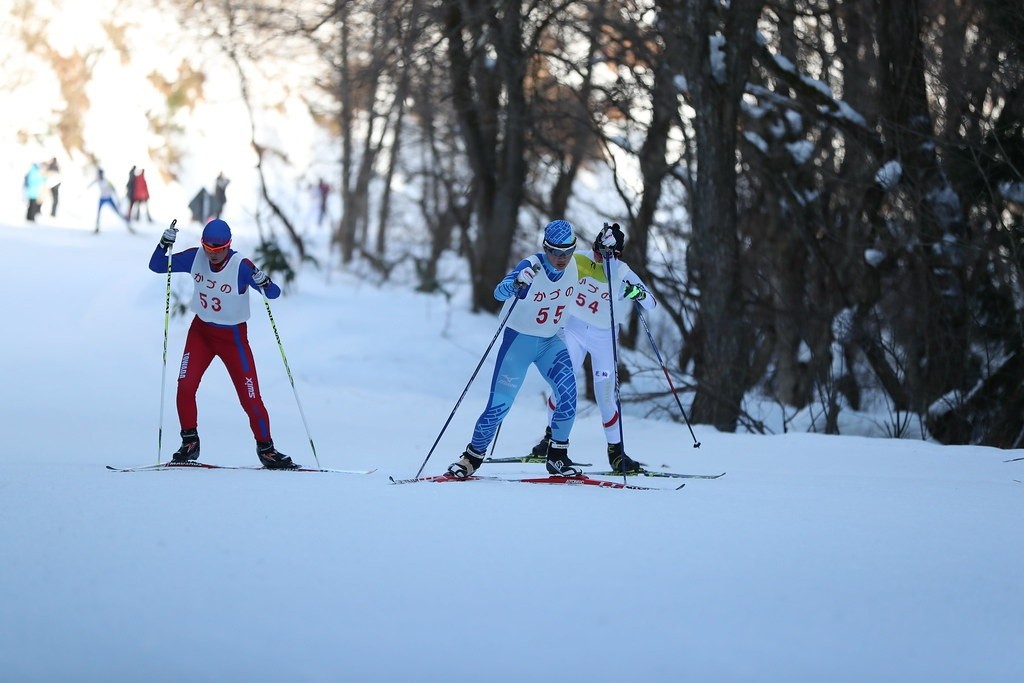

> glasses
xmin=201 ymin=241 xmax=229 ymax=254
xmin=545 ymin=237 xmax=577 ymax=257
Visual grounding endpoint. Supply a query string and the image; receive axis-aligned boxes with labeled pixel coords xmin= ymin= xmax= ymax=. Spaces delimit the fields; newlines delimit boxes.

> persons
xmin=25 ymin=162 xmax=46 ymax=222
xmin=149 ymin=219 xmax=298 ymax=469
xmin=47 ymin=158 xmax=61 ymax=216
xmin=125 ymin=165 xmax=152 ymax=221
xmin=444 ymin=220 xmax=618 ymax=481
xmin=214 ymin=173 xmax=230 ymax=220
xmin=531 ymin=223 xmax=656 ymax=475
xmin=87 ymin=169 xmax=126 ymax=234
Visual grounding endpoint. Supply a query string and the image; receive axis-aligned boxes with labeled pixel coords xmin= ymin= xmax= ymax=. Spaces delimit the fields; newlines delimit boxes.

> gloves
xmin=624 ymin=285 xmax=641 ymax=300
xmin=601 ymin=228 xmax=617 ymax=248
xmin=514 ymin=267 xmax=535 ymax=286
xmin=252 ymin=271 xmax=268 ymax=286
xmin=160 ymin=228 xmax=179 ymax=248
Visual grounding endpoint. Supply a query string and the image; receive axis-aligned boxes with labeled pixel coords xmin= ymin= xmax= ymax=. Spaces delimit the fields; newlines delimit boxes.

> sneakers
xmin=546 ymin=439 xmax=582 ymax=476
xmin=256 ymin=441 xmax=291 ymax=463
xmin=173 ymin=429 xmax=200 ymax=461
xmin=532 ymin=426 xmax=552 ymax=455
xmin=448 ymin=443 xmax=487 ymax=477
xmin=606 ymin=443 xmax=639 ymax=471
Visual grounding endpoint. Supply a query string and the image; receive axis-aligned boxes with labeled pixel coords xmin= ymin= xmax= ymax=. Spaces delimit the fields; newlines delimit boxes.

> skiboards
xmin=105 ymin=427 xmax=378 ymax=477
xmin=388 ymin=436 xmax=686 ymax=492
xmin=480 ymin=424 xmax=726 ymax=480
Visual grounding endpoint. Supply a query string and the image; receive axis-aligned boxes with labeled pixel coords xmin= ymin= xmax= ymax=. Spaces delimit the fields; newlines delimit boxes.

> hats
xmin=595 ymin=223 xmax=624 ymax=252
xmin=544 ymin=220 xmax=575 ymax=245
xmin=203 ymin=219 xmax=231 ymax=244
